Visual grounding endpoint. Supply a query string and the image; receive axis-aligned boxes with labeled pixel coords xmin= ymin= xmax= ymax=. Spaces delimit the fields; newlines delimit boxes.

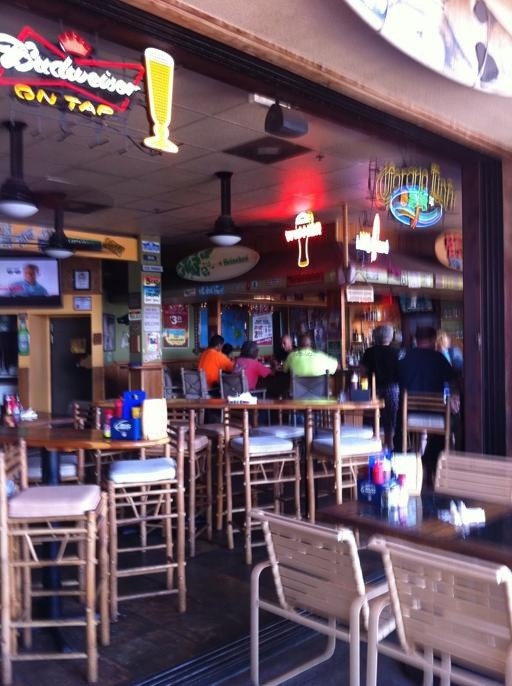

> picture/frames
xmin=72 ymin=269 xmax=92 ymax=290
xmin=103 ymin=312 xmax=117 ymax=352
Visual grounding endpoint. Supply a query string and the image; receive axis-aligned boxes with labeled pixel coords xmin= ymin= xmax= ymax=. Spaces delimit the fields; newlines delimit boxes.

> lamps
xmin=1 ymin=120 xmax=39 ymax=219
xmin=207 ymin=169 xmax=243 ymax=248
xmin=261 ymin=81 xmax=312 ymax=138
xmin=42 ymin=208 xmax=74 ymax=258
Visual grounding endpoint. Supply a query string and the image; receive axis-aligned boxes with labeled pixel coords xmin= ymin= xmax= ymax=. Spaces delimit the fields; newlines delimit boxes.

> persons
xmin=390 ymin=327 xmax=459 ymax=489
xmin=194 ymin=320 xmax=465 ymax=453
xmin=8 ymin=259 xmax=52 ymax=297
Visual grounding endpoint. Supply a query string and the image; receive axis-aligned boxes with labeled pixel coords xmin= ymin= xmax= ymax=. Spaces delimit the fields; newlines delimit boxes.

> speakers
xmin=264 ymin=104 xmax=309 ymax=139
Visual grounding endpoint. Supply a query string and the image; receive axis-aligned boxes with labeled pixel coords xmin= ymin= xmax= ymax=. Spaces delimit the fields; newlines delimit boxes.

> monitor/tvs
xmin=397 ymin=295 xmax=435 ymax=316
xmin=1 ymin=249 xmax=63 ymax=310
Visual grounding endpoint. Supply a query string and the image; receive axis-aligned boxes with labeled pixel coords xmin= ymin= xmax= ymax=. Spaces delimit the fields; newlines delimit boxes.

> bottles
xmin=373 ymin=461 xmax=473 ymax=530
xmin=18 ymin=319 xmax=31 ymax=356
xmin=5 ymin=393 xmax=122 ymax=440
